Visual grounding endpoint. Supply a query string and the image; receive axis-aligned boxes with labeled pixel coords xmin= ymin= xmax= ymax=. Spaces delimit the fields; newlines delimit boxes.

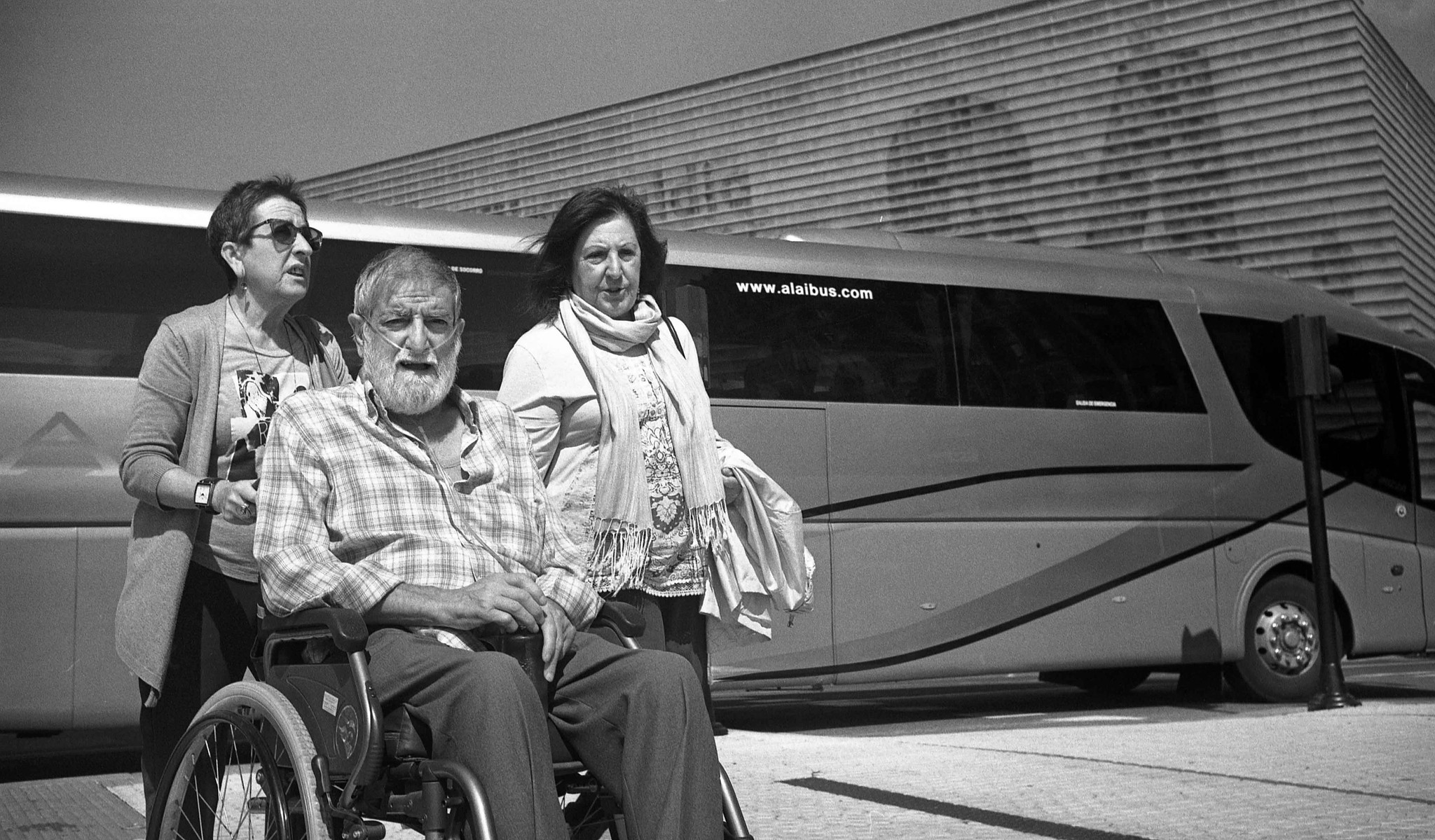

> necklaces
xmin=227 ymin=293 xmax=297 ymax=411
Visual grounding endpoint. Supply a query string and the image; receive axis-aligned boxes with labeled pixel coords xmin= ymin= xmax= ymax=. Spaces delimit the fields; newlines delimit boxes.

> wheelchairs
xmin=143 ymin=478 xmax=756 ymax=840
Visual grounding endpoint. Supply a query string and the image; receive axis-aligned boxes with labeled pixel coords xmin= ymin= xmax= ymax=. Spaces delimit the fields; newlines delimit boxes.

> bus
xmin=0 ymin=170 xmax=1435 ymax=734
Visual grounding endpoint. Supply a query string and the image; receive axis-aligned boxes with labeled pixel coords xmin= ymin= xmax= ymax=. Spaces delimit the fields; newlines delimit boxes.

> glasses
xmin=237 ymin=217 xmax=324 ymax=250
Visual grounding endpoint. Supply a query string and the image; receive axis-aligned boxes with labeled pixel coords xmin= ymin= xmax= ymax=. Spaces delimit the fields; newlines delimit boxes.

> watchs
xmin=193 ymin=478 xmax=226 ymax=515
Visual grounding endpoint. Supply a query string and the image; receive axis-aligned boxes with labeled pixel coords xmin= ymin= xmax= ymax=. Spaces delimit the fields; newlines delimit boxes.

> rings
xmin=240 ymin=501 xmax=249 ymax=515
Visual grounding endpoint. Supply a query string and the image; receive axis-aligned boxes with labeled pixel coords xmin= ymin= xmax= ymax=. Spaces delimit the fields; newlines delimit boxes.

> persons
xmin=251 ymin=243 xmax=726 ymax=840
xmin=492 ymin=186 xmax=742 ymax=840
xmin=111 ymin=173 xmax=355 ymax=840
